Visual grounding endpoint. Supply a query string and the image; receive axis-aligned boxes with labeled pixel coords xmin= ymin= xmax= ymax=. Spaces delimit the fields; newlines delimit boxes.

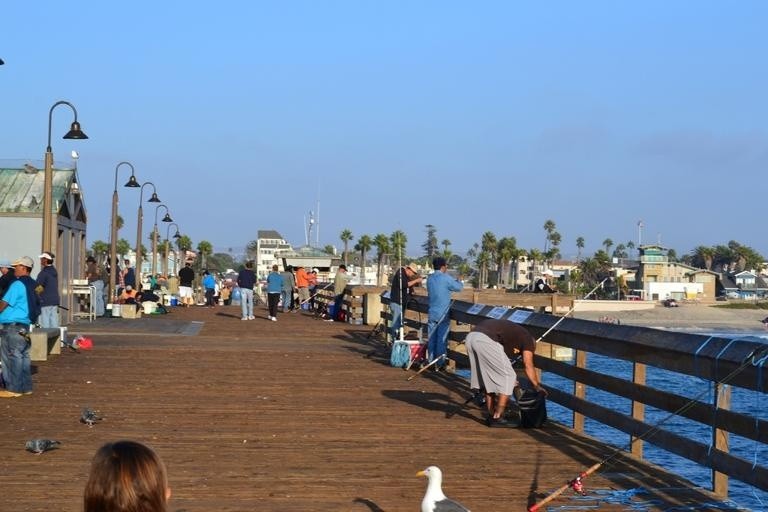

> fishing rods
xmin=407 ymin=338 xmax=466 ymax=381
xmin=300 ymin=280 xmax=334 ymax=305
xmin=445 ymin=276 xmax=609 ymax=418
xmin=529 ymin=346 xmax=766 ymax=512
xmin=406 ymin=269 xmax=493 ymax=371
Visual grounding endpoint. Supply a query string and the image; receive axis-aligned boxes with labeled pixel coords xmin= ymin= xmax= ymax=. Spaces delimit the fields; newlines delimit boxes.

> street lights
xmin=105 ymin=160 xmax=143 ymax=305
xmin=133 ymin=179 xmax=162 ymax=294
xmin=1 ymin=59 xmax=5 ymax=69
xmin=151 ymin=203 xmax=188 ymax=282
xmin=42 ymin=97 xmax=91 ymax=279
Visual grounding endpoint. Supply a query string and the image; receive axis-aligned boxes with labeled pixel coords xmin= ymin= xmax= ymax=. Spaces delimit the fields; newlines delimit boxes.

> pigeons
xmin=80 ymin=409 xmax=108 ymax=429
xmin=24 ymin=439 xmax=61 ymax=455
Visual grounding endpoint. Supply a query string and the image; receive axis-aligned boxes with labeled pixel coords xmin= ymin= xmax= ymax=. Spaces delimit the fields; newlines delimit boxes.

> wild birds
xmin=415 ymin=466 xmax=470 ymax=512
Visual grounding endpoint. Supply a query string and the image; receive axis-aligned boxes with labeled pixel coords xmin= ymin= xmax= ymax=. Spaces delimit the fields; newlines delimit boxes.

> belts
xmin=0 ymin=322 xmax=28 ymax=328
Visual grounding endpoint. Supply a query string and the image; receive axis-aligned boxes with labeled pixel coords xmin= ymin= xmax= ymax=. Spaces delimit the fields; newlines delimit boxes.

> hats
xmin=405 ymin=262 xmax=419 ymax=275
xmin=0 ymin=259 xmax=14 ymax=268
xmin=37 ymin=252 xmax=54 ymax=262
xmin=125 ymin=285 xmax=132 ymax=292
xmin=339 ymin=264 xmax=346 ymax=271
xmin=10 ymin=255 xmax=34 ymax=269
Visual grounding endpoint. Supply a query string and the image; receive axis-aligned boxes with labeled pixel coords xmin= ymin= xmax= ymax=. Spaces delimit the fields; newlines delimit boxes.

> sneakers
xmin=268 ymin=315 xmax=278 ymax=322
xmin=426 ymin=363 xmax=455 ymax=373
xmin=484 ymin=411 xmax=519 ymax=430
xmin=240 ymin=316 xmax=256 ymax=321
xmin=0 ymin=389 xmax=33 ymax=399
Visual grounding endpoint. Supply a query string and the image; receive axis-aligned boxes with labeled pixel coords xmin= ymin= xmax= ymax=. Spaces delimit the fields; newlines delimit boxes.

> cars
xmin=664 ymin=298 xmax=679 ymax=307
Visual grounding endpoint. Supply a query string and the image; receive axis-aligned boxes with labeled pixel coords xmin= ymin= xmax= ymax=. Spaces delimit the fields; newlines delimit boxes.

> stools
xmin=29 ymin=328 xmax=60 ymax=361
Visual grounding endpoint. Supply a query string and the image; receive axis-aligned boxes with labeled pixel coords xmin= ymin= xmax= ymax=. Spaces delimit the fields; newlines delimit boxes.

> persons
xmin=0 ymin=251 xmax=60 ymax=398
xmin=83 ymin=439 xmax=171 ymax=511
xmin=84 ymin=256 xmax=350 ymax=323
xmin=465 ymin=319 xmax=548 ymax=428
xmin=426 ymin=257 xmax=464 ymax=372
xmin=388 ymin=257 xmax=423 ymax=344
